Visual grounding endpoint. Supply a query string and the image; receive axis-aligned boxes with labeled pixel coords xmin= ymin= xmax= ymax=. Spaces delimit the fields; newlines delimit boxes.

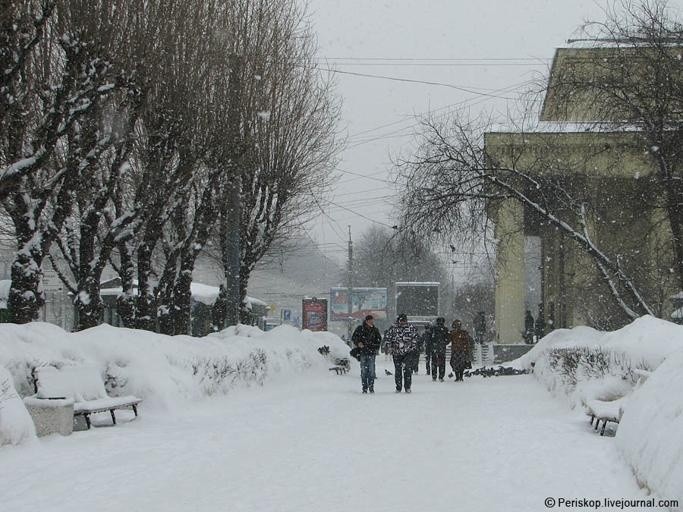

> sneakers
xmin=360 ymin=371 xmax=464 ymax=394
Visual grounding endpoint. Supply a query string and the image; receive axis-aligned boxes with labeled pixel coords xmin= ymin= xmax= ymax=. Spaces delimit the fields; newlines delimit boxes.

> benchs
xmin=583 ymin=392 xmax=634 ymax=435
xmin=32 ymin=362 xmax=140 ymax=428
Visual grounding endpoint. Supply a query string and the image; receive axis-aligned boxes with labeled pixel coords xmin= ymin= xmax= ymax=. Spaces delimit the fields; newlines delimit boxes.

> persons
xmin=349 ymin=302 xmax=554 ymax=395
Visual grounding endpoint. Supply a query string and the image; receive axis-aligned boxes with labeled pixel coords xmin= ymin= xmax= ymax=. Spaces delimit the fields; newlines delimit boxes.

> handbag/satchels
xmin=350 ymin=347 xmax=361 ymax=362
